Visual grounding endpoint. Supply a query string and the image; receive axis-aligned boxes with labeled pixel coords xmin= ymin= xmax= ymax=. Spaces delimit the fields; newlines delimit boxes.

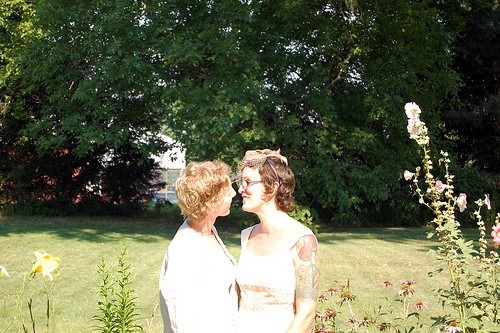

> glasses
xmin=234 ymin=178 xmax=265 ymax=190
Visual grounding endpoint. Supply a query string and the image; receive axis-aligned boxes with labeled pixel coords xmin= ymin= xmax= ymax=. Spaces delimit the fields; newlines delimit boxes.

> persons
xmin=160 ymin=158 xmax=238 ymax=333
xmin=235 ymin=148 xmax=319 ymax=333
